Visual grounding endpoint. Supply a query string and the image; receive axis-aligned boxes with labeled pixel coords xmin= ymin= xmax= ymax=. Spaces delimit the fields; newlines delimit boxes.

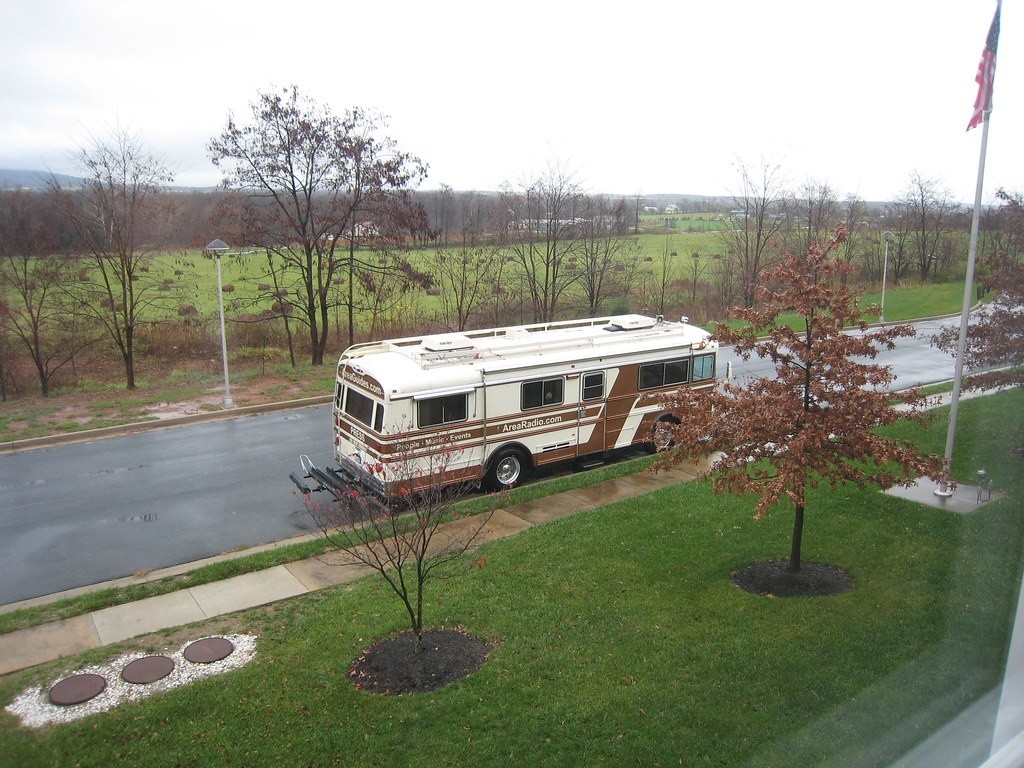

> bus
xmin=289 ymin=310 xmax=733 ymax=516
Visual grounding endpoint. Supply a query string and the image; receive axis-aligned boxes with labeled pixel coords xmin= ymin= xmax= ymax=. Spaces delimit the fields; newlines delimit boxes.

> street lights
xmin=877 ymin=231 xmax=895 ymax=323
xmin=206 ymin=238 xmax=234 ymax=410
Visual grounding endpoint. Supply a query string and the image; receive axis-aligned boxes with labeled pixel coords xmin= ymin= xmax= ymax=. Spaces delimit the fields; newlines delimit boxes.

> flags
xmin=966 ymin=5 xmax=1001 ymax=131
